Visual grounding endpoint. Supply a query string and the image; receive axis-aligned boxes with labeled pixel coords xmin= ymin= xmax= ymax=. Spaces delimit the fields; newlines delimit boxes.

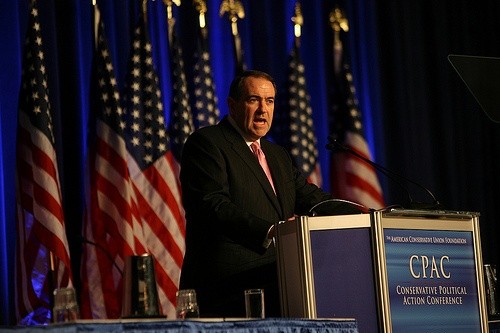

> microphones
xmin=77 ymin=234 xmax=123 ymax=276
xmin=327 ymin=134 xmax=440 ymax=209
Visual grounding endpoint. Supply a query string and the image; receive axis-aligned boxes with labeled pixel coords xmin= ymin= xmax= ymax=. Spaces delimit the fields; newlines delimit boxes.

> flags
xmin=185 ymin=0 xmax=220 ymax=131
xmin=18 ymin=0 xmax=74 ymax=328
xmin=216 ymin=0 xmax=251 ymax=72
xmin=82 ymin=0 xmax=147 ymax=320
xmin=284 ymin=0 xmax=323 ymax=192
xmin=127 ymin=0 xmax=188 ymax=319
xmin=320 ymin=0 xmax=385 ymax=213
xmin=163 ymin=0 xmax=195 ymax=151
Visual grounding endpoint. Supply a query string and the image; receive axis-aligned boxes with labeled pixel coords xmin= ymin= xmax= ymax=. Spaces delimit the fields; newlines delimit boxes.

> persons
xmin=180 ymin=68 xmax=376 ymax=319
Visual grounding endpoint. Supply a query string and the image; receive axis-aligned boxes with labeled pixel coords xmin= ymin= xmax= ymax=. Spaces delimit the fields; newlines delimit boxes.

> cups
xmin=175 ymin=289 xmax=200 ymax=320
xmin=245 ymin=289 xmax=265 ymax=320
xmin=53 ymin=288 xmax=81 ymax=324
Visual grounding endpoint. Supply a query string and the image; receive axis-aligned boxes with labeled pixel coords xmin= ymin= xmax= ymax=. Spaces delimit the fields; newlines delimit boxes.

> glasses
xmin=250 ymin=140 xmax=273 ymax=185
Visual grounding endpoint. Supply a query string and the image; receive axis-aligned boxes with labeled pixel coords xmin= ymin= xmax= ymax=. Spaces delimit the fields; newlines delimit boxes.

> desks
xmin=0 ymin=318 xmax=357 ymax=333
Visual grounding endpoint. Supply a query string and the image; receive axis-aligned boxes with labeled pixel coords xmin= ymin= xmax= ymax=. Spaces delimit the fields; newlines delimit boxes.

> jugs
xmin=482 ymin=264 xmax=500 ymax=320
xmin=123 ymin=253 xmax=159 ymax=318
